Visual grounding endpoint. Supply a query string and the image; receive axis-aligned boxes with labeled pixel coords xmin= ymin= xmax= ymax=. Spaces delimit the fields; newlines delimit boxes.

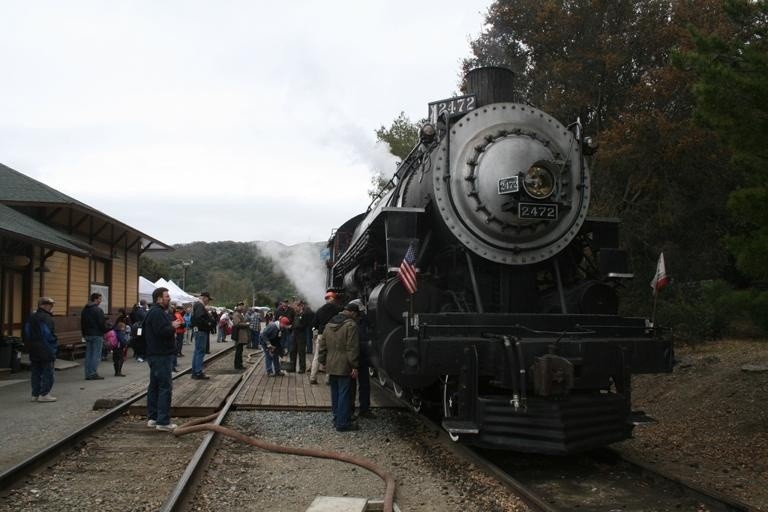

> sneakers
xmin=37 ymin=393 xmax=57 ymax=403
xmin=234 ymin=364 xmax=306 ymax=378
xmin=311 ymin=380 xmax=318 ymax=384
xmin=173 ymin=352 xmax=185 ymax=373
xmin=156 ymin=423 xmax=178 ymax=432
xmin=192 ymin=373 xmax=209 ymax=380
xmin=334 ymin=410 xmax=378 ymax=432
xmin=31 ymin=396 xmax=38 ymax=401
xmin=146 ymin=419 xmax=157 ymax=427
xmin=114 ymin=357 xmax=144 ymax=378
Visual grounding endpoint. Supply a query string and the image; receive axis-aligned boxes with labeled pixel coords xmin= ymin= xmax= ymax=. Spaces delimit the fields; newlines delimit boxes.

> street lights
xmin=178 ymin=257 xmax=194 ymax=293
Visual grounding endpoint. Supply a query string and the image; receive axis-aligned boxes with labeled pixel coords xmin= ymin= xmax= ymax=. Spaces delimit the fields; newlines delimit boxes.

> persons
xmin=310 ymin=289 xmax=377 ymax=432
xmin=23 ymin=297 xmax=59 ymax=401
xmin=165 ymin=302 xmax=194 ymax=372
xmin=100 ymin=299 xmax=152 ymax=376
xmin=205 ymin=295 xmax=327 ymax=376
xmin=191 ymin=291 xmax=214 ymax=380
xmin=142 ymin=288 xmax=182 ymax=432
xmin=80 ymin=293 xmax=108 ymax=381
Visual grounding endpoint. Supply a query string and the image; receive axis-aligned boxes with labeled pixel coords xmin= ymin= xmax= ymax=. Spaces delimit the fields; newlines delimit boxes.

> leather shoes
xmin=86 ymin=374 xmax=105 ymax=380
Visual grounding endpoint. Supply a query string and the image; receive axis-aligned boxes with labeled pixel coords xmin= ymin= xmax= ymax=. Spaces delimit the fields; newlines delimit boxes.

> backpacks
xmin=104 ymin=329 xmax=120 ymax=349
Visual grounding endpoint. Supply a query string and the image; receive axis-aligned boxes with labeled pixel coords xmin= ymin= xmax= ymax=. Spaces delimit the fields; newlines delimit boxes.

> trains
xmin=322 ymin=65 xmax=676 ymax=461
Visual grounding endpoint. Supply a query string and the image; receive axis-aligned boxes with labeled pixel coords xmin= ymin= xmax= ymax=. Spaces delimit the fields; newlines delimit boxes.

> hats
xmin=237 ymin=302 xmax=245 ymax=307
xmin=38 ymin=296 xmax=56 ymax=305
xmin=324 ymin=292 xmax=337 ymax=300
xmin=344 ymin=298 xmax=366 ymax=312
xmin=200 ymin=291 xmax=215 ymax=300
xmin=279 ymin=316 xmax=290 ymax=327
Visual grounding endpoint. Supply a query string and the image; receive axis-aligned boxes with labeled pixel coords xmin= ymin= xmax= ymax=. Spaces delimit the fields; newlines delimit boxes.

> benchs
xmin=53 ymin=315 xmax=120 ymax=361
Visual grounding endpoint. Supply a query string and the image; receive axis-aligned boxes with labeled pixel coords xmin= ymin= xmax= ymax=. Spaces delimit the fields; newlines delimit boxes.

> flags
xmin=648 ymin=252 xmax=672 ymax=299
xmin=395 ymin=240 xmax=419 ymax=298
